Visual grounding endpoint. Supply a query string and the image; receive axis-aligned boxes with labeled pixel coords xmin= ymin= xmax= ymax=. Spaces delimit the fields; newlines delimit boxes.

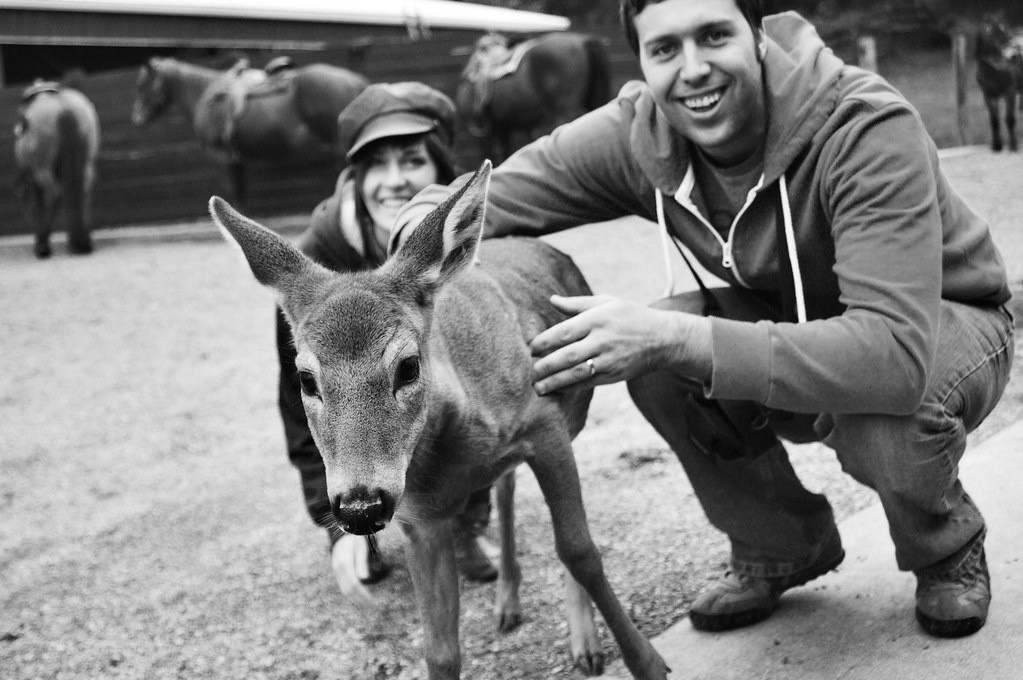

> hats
xmin=338 ymin=81 xmax=457 ymax=162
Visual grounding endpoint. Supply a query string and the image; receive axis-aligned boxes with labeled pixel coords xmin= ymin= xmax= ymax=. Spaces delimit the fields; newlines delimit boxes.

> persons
xmin=388 ymin=0 xmax=1016 ymax=638
xmin=276 ymin=82 xmax=502 ymax=609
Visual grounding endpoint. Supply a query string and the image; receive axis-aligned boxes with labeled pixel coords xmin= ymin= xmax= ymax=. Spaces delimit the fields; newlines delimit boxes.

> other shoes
xmin=328 ymin=527 xmax=385 ymax=585
xmin=455 ymin=534 xmax=501 ymax=583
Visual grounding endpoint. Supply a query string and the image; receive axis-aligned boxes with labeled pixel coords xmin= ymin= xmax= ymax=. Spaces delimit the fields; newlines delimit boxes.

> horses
xmin=133 ymin=34 xmax=614 ymax=223
xmin=15 ymin=80 xmax=102 ymax=259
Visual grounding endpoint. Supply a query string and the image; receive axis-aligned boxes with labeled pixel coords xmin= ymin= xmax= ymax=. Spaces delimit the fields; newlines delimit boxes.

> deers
xmin=209 ymin=158 xmax=674 ymax=680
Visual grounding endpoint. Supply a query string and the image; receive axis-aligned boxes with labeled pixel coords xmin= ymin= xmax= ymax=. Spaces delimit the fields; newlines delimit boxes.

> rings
xmin=587 ymin=359 xmax=595 ymax=377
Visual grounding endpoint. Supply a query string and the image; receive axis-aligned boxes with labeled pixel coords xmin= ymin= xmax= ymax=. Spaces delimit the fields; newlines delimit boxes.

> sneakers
xmin=688 ymin=525 xmax=844 ymax=628
xmin=914 ymin=547 xmax=990 ymax=638
